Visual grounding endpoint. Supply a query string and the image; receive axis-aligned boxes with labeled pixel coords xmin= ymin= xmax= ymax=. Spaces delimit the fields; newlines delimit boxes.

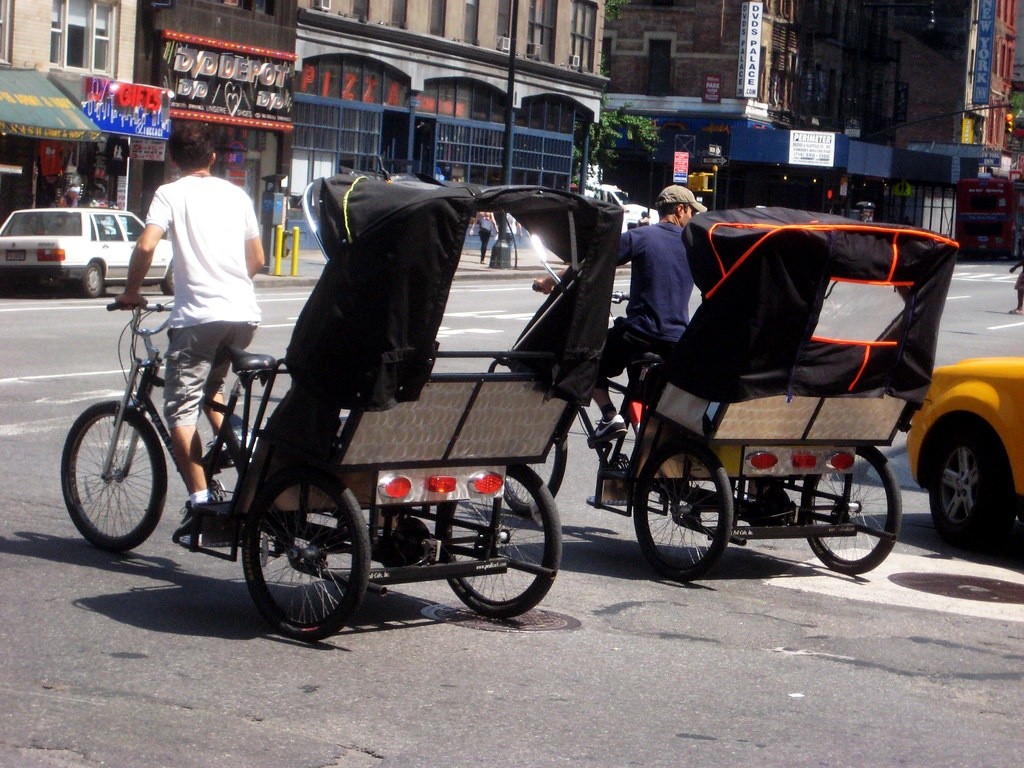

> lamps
xmin=164 ymin=91 xmax=175 ymax=100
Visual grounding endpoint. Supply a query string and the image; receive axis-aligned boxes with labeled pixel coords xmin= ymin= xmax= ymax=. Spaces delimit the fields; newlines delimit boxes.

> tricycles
xmin=529 ymin=204 xmax=960 ymax=586
xmin=58 ymin=154 xmax=625 ymax=643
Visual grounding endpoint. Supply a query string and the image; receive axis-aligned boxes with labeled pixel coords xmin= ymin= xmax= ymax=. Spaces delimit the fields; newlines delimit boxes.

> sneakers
xmin=202 ymin=439 xmax=236 ymax=468
xmin=588 ymin=413 xmax=627 ymax=446
xmin=172 ymin=497 xmax=217 ymax=543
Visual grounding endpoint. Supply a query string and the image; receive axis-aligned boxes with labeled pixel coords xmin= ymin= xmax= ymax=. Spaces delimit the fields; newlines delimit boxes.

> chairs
xmin=20 ymin=215 xmax=45 ymax=235
xmin=94 ymin=223 xmax=111 ymax=241
xmin=52 ymin=216 xmax=83 ymax=236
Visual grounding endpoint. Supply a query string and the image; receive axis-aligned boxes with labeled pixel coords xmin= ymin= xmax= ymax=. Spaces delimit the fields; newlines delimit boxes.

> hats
xmin=656 ymin=186 xmax=708 ymax=213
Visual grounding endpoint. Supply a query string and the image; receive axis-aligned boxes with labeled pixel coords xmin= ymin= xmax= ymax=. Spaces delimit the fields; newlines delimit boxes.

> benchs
xmin=676 ymin=332 xmax=920 ymax=446
xmin=266 ymin=347 xmax=589 ymax=471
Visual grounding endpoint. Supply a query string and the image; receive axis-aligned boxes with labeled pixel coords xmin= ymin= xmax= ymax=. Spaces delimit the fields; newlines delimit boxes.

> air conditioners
xmin=311 ymin=0 xmax=331 ymax=11
xmin=526 ymin=43 xmax=543 ymax=58
xmin=569 ymin=56 xmax=580 ymax=68
xmin=496 ymin=36 xmax=510 ymax=51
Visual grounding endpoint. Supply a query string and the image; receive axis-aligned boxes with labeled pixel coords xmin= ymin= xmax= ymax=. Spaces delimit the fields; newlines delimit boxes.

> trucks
xmin=953 ymin=173 xmax=1024 ymax=261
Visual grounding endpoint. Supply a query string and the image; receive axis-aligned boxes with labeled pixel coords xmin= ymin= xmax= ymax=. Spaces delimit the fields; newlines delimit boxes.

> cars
xmin=905 ymin=355 xmax=1024 ymax=551
xmin=0 ymin=207 xmax=175 ymax=299
xmin=583 ymin=183 xmax=660 ymax=233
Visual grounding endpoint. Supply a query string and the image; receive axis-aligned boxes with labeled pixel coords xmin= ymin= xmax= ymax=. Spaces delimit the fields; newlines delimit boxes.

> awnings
xmin=0 ymin=69 xmax=102 ymax=143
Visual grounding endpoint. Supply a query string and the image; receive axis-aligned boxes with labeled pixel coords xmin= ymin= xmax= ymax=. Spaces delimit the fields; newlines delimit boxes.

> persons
xmin=114 ymin=122 xmax=265 ymax=544
xmin=60 ymin=184 xmax=81 ymax=208
xmin=537 ymin=185 xmax=708 ymax=443
xmin=506 ymin=213 xmax=522 ymax=249
xmin=470 ymin=212 xmax=499 ymax=264
xmin=1009 ymin=226 xmax=1024 ymax=315
xmin=637 ymin=212 xmax=650 ymax=227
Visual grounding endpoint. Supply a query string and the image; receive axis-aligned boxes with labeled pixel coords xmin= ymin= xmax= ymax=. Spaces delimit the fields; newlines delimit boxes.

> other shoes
xmin=1009 ymin=308 xmax=1024 ymax=314
